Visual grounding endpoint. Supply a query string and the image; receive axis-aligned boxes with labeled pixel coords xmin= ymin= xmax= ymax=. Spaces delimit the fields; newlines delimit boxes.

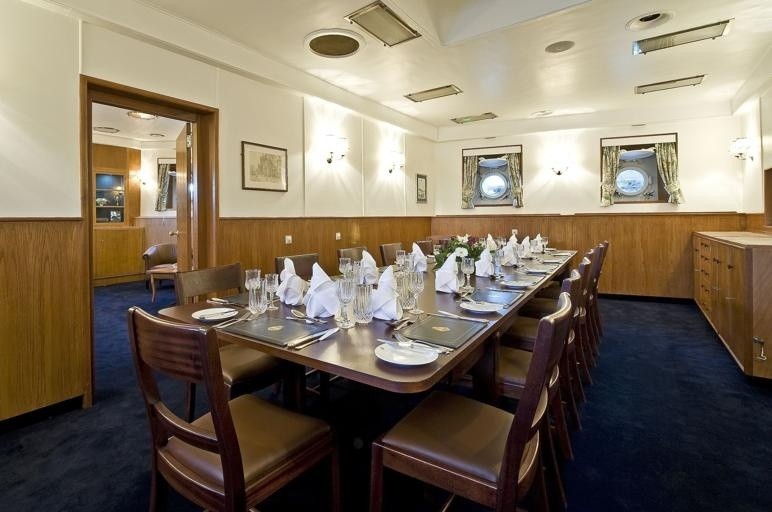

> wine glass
xmin=264 ymin=272 xmax=279 ymax=311
xmin=462 ymin=257 xmax=475 ymax=292
xmin=478 ymin=236 xmax=550 ymax=278
xmin=334 ymin=249 xmax=426 ymax=326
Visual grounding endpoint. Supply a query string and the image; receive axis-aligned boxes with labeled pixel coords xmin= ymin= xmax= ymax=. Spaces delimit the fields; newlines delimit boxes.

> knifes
xmin=436 ymin=310 xmax=490 ymax=323
xmin=286 ymin=327 xmax=339 ymax=351
xmin=196 ymin=309 xmax=242 ymax=322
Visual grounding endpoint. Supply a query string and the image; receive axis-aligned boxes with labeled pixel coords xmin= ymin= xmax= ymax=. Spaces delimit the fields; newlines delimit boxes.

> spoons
xmin=290 ymin=309 xmax=328 ymax=326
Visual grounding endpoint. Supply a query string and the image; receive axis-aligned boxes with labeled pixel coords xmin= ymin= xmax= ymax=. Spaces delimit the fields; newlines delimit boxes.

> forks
xmin=219 ymin=311 xmax=252 ymax=330
xmin=394 ymin=332 xmax=455 ymax=353
xmin=285 ymin=317 xmax=314 ymax=324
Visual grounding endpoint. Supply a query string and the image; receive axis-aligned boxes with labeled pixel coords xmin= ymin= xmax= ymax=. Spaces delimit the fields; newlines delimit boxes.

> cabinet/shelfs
xmin=692 ymin=235 xmax=772 ymax=381
xmin=95 ymin=172 xmax=125 ymax=223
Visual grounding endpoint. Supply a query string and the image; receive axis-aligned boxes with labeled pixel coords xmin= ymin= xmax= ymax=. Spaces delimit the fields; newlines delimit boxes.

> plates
xmin=191 ymin=307 xmax=243 ymax=325
xmin=553 ymin=253 xmax=571 ymax=255
xmin=500 ymin=280 xmax=530 ymax=289
xmin=544 ymin=259 xmax=559 ymax=263
xmin=527 ymin=269 xmax=548 ymax=274
xmin=460 ymin=301 xmax=502 ymax=317
xmin=369 ymin=341 xmax=439 ymax=369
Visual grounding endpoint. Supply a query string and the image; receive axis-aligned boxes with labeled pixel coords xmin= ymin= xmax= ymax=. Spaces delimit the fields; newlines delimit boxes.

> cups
xmin=243 ymin=268 xmax=268 ymax=316
xmin=433 ymin=245 xmax=441 ymax=255
xmin=453 ymin=262 xmax=466 ymax=288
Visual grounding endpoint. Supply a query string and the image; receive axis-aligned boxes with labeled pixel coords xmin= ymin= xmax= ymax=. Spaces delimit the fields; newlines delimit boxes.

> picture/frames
xmin=241 ymin=141 xmax=288 ymax=191
xmin=416 ymin=173 xmax=427 ymax=203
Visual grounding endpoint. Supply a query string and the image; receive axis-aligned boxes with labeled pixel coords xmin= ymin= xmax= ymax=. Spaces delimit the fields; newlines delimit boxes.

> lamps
xmin=730 ymin=138 xmax=753 ymax=160
xmin=326 ymin=134 xmax=348 ymax=163
